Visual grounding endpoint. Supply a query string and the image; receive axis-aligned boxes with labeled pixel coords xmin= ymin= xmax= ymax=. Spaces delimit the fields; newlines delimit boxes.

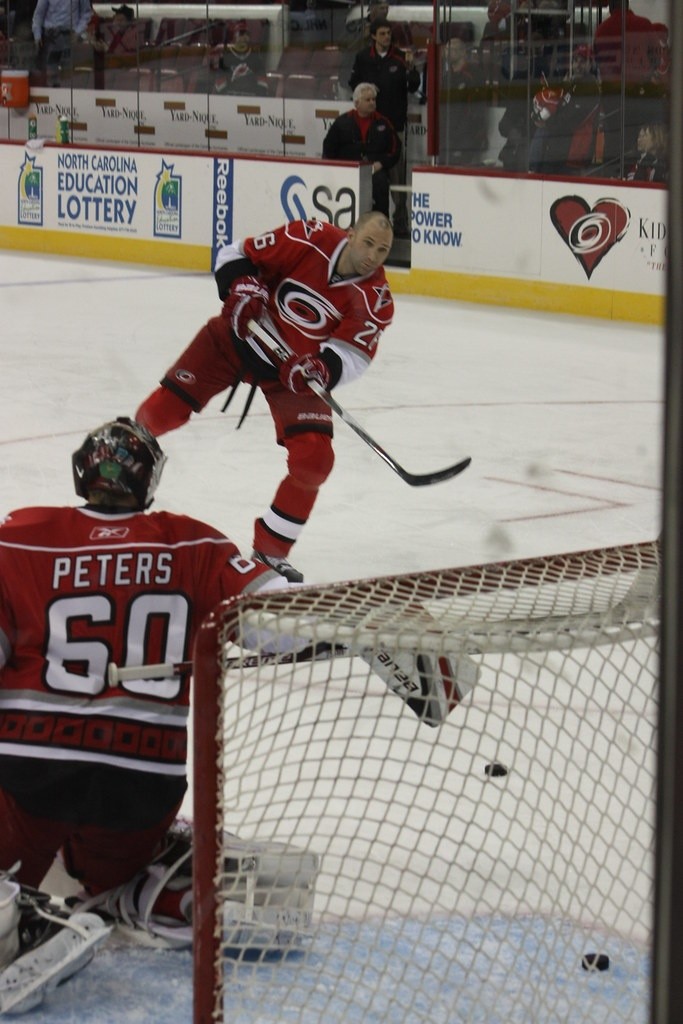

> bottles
xmin=56 ymin=114 xmax=69 ymax=144
xmin=28 ymin=112 xmax=38 ymax=140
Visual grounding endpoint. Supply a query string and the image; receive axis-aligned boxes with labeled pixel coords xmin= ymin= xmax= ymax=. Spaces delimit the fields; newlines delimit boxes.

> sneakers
xmin=251 ymin=550 xmax=304 ymax=587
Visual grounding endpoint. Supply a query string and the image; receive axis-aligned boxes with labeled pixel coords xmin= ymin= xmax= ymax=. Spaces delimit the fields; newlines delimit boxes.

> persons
xmin=212 ymin=25 xmax=269 ymax=95
xmin=323 ymin=83 xmax=402 ymax=217
xmin=347 ymin=21 xmax=420 ymax=237
xmin=0 ymin=415 xmax=320 ymax=961
xmin=137 ymin=210 xmax=394 ymax=585
xmin=32 ymin=0 xmax=134 ymax=86
xmin=438 ymin=1 xmax=672 ymax=185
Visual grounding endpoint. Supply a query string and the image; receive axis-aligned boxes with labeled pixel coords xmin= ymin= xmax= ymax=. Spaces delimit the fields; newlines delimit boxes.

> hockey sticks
xmin=106 ymin=568 xmax=659 ymax=689
xmin=239 ymin=313 xmax=471 ymax=487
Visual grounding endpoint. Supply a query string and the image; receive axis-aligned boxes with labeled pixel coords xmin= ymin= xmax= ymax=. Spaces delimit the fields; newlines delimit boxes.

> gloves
xmin=226 ymin=274 xmax=268 ymax=340
xmin=280 ymin=352 xmax=331 ymax=396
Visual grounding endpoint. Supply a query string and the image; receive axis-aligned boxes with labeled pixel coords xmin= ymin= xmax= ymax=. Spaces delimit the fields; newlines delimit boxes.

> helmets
xmin=71 ymin=417 xmax=167 ymax=508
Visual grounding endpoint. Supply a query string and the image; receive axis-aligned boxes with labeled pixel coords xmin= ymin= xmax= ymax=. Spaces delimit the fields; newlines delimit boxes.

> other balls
xmin=581 ymin=954 xmax=612 ymax=974
xmin=483 ymin=763 xmax=508 ymax=778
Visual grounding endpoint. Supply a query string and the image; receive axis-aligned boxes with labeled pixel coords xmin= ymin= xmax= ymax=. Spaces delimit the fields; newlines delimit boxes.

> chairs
xmin=71 ymin=20 xmax=586 ymax=100
